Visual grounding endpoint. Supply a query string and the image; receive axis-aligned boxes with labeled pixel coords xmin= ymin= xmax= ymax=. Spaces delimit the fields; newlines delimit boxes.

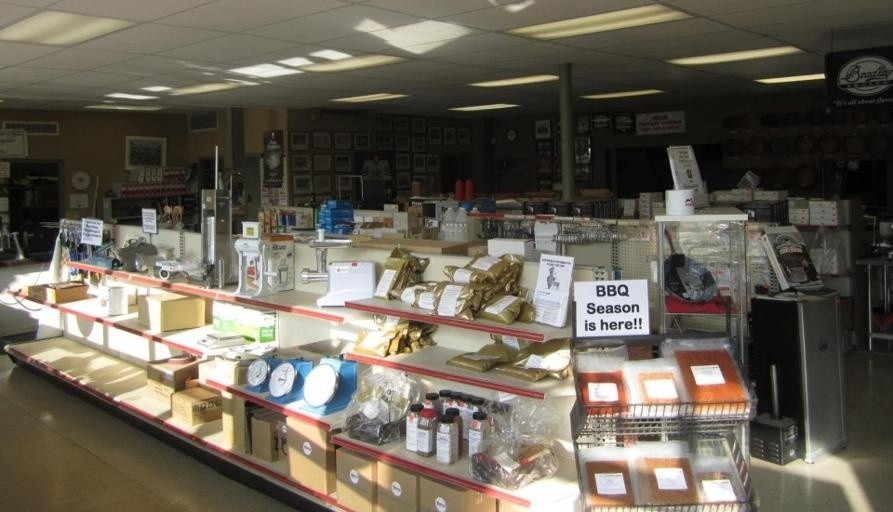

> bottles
xmin=405 ymin=389 xmax=511 ymax=467
xmin=439 ymin=206 xmax=481 ymax=242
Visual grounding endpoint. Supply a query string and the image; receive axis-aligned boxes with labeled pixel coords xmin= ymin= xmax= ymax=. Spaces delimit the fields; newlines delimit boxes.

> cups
xmin=665 ymin=190 xmax=695 ymax=216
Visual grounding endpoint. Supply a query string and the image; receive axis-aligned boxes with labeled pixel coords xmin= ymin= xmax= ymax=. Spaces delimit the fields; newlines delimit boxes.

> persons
xmin=360 ymin=150 xmax=390 ymax=169
xmin=183 ymin=160 xmax=206 ymax=229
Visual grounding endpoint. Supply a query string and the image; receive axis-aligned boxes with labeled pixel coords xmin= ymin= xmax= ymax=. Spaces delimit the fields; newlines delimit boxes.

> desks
xmin=856 ymin=246 xmax=893 ymax=353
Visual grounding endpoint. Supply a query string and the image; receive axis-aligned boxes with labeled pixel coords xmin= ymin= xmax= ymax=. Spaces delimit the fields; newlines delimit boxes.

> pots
xmin=663 ymin=223 xmax=718 ymax=302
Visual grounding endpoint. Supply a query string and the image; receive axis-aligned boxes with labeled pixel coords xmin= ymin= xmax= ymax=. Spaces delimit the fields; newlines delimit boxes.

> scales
xmin=246 ymin=354 xmax=358 ymax=414
xmin=196 ymin=333 xmax=246 ymax=347
